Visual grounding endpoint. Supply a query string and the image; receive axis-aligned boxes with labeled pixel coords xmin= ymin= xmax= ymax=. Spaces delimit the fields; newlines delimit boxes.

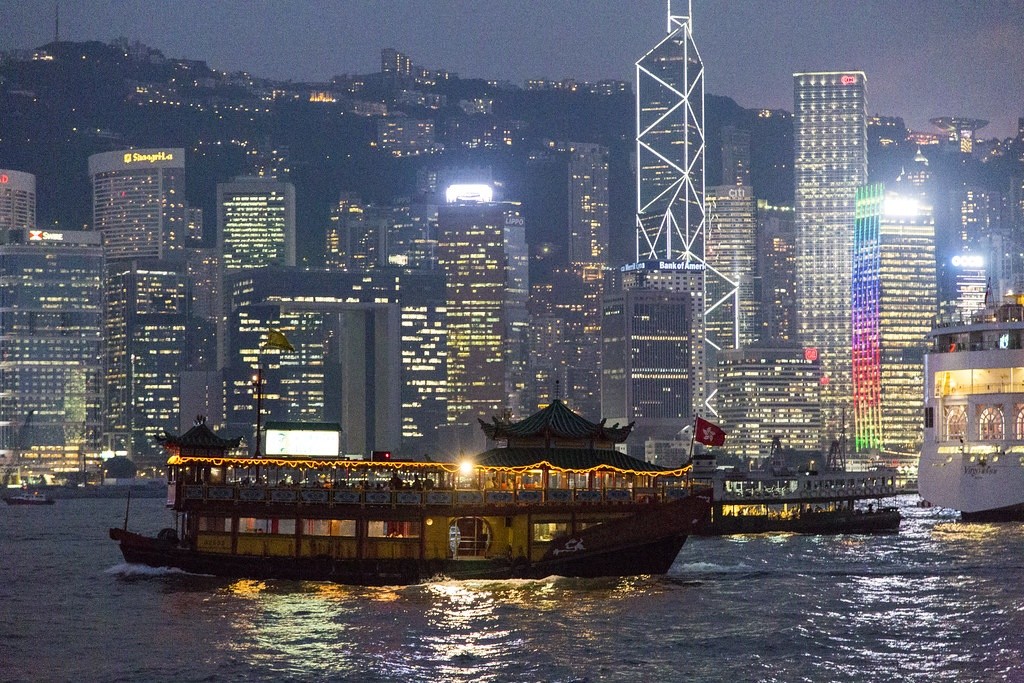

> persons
xmin=484 ymin=477 xmax=568 ymax=488
xmin=412 ymin=474 xmax=421 ymax=489
xmin=390 ymin=473 xmax=403 ymax=489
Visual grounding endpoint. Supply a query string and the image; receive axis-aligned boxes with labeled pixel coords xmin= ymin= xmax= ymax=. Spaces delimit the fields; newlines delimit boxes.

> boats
xmin=108 ymin=365 xmax=714 ymax=594
xmin=3 ymin=491 xmax=56 ymax=506
xmin=915 ymin=275 xmax=1024 ymax=524
xmin=692 ymin=403 xmax=906 ymax=537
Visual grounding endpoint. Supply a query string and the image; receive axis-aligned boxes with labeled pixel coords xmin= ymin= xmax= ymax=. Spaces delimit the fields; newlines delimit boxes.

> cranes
xmin=0 ymin=409 xmax=35 ymax=500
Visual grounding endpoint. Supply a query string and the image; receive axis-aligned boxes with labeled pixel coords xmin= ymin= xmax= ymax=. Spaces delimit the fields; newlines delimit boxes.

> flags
xmin=261 ymin=330 xmax=294 ymax=352
xmin=985 ymin=278 xmax=992 ymax=304
xmin=694 ymin=417 xmax=727 ymax=447
